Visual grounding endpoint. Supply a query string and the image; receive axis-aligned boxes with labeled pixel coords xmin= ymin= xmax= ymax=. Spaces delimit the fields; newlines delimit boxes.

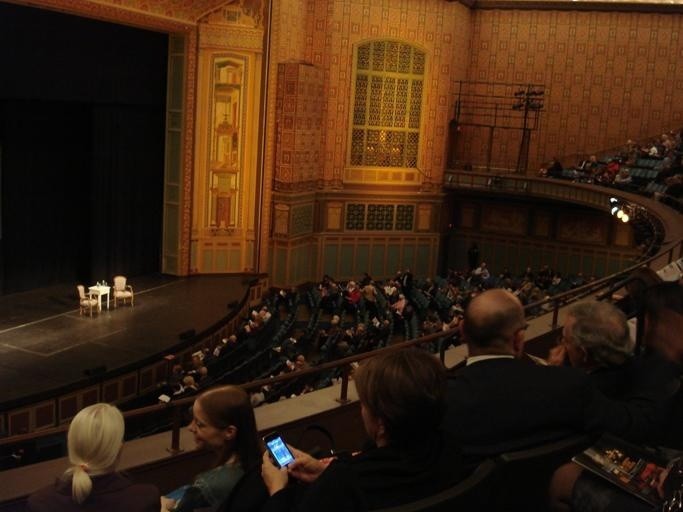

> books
xmin=568 ymin=435 xmax=682 ymax=507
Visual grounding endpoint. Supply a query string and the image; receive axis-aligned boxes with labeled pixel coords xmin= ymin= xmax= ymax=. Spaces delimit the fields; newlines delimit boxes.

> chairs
xmin=112 ymin=275 xmax=134 ymax=308
xmin=557 ymin=129 xmax=682 ymax=204
xmin=76 ymin=284 xmax=98 ymax=317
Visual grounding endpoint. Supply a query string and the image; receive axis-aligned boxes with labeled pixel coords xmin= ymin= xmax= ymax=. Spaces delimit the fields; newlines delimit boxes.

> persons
xmin=23 ymin=399 xmax=161 ymax=512
xmin=156 ymin=260 xmax=683 ymax=411
xmin=548 ymin=441 xmax=683 ymax=511
xmin=544 ymin=299 xmax=682 ymax=449
xmin=157 ymin=384 xmax=270 ymax=511
xmin=259 ymin=343 xmax=475 ymax=512
xmin=444 ymin=286 xmax=587 ymax=462
xmin=535 ymin=127 xmax=682 ymax=210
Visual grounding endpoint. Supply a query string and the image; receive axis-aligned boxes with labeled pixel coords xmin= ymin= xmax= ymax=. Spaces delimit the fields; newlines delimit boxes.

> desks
xmin=88 ymin=285 xmax=111 ymax=311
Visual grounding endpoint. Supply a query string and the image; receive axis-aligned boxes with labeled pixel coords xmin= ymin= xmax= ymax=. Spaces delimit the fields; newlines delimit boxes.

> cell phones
xmin=262 ymin=430 xmax=296 ymax=468
